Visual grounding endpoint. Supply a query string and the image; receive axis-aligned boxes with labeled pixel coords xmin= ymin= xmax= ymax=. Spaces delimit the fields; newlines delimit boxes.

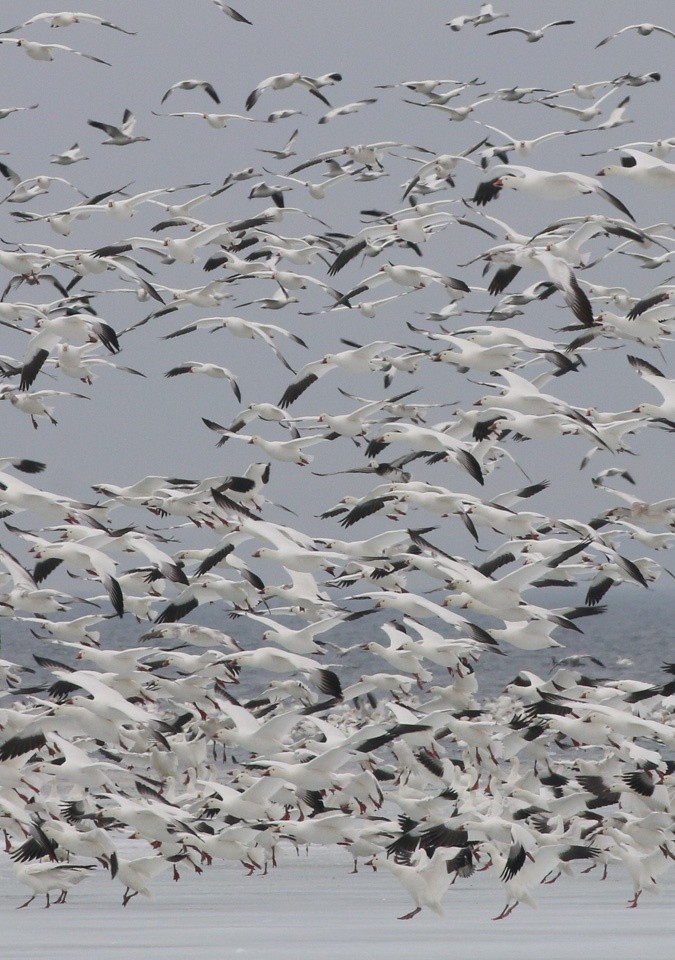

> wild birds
xmin=0 ymin=0 xmax=675 ymax=922
